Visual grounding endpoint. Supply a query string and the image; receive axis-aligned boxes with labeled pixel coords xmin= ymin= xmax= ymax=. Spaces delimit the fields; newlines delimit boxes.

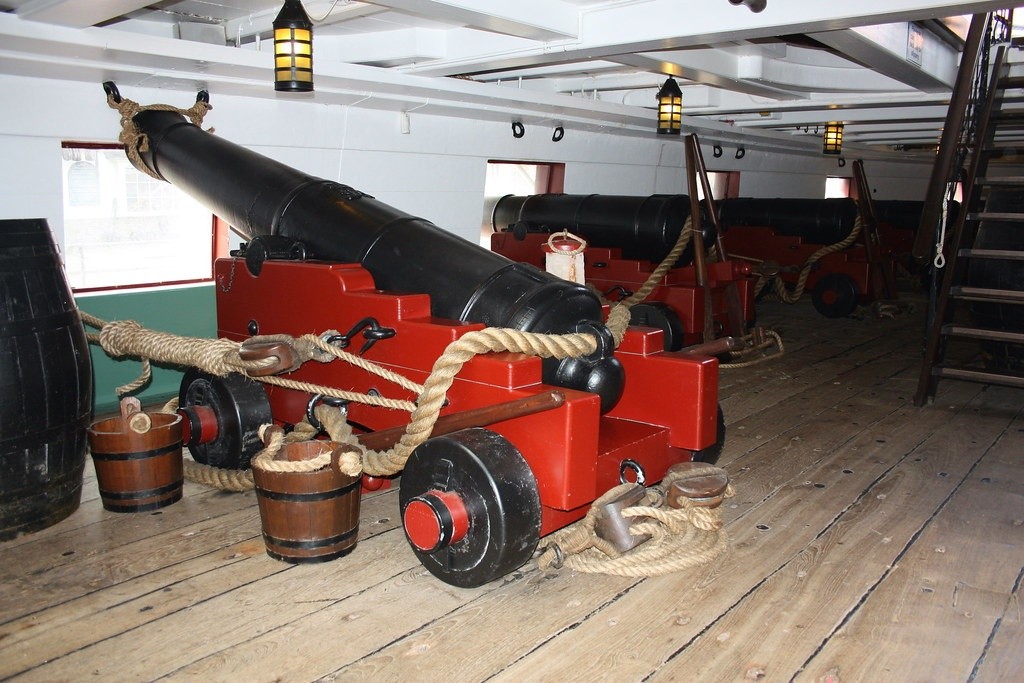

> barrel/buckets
xmin=86 ymin=395 xmax=186 ymax=514
xmin=250 ymin=424 xmax=365 ymax=567
xmin=1 ymin=216 xmax=93 ymax=540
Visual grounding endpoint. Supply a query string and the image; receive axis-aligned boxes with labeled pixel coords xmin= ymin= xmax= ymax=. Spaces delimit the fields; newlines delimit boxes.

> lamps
xmin=657 ymin=74 xmax=683 ymax=135
xmin=272 ymin=0 xmax=315 ymax=92
xmin=822 ymin=124 xmax=844 ymax=156
